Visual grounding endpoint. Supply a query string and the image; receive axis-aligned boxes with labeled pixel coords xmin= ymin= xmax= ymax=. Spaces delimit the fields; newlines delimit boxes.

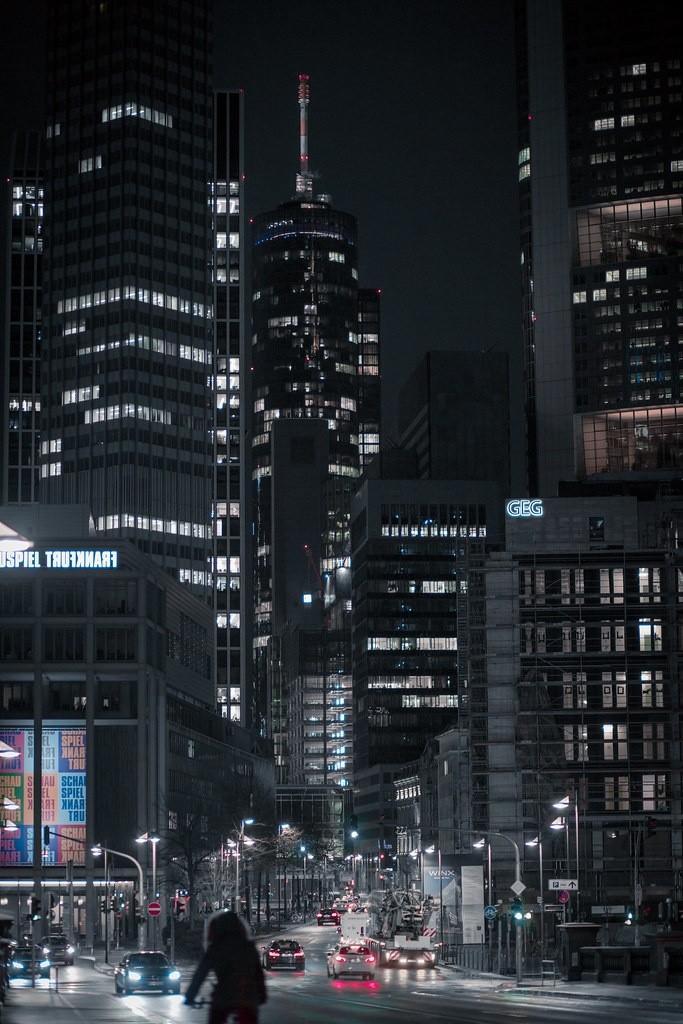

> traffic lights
xmin=379 ymin=851 xmax=386 ymax=870
xmin=177 ymin=901 xmax=185 ymax=915
xmin=511 ymin=897 xmax=523 ymax=925
xmin=623 ymin=901 xmax=635 ymax=927
xmin=110 ymin=897 xmax=117 ymax=912
xmin=31 ymin=897 xmax=42 ymax=921
xmin=100 ymin=900 xmax=106 ymax=914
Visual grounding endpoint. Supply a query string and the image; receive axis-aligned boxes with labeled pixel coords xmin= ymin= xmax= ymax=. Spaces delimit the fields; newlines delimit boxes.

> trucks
xmin=340 ymin=889 xmax=443 ymax=968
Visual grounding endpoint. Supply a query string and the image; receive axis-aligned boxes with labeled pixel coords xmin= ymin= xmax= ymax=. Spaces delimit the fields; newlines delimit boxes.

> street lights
xmin=0 ymin=884 xmax=33 ymax=945
xmin=473 ymin=838 xmax=494 ymax=973
xmin=238 ymin=817 xmax=254 ymax=889
xmin=425 ymin=843 xmax=444 ymax=961
xmin=134 ymin=830 xmax=161 ymax=950
xmin=91 ymin=840 xmax=108 ymax=964
xmin=278 ymin=823 xmax=290 ymax=927
xmin=525 ymin=791 xmax=580 ymax=978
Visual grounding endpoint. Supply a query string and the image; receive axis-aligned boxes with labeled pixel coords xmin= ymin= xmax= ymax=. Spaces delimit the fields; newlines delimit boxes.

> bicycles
xmin=248 ymin=908 xmax=318 ymax=936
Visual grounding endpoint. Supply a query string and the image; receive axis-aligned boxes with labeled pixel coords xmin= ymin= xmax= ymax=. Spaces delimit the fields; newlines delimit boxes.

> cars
xmin=9 ymin=935 xmax=74 ymax=979
xmin=261 ymin=940 xmax=305 ymax=971
xmin=326 ymin=934 xmax=377 ymax=980
xmin=316 ymin=889 xmax=368 ymax=926
xmin=114 ymin=951 xmax=181 ymax=995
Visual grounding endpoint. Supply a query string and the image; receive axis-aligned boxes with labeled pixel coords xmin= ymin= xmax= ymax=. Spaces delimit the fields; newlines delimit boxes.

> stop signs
xmin=147 ymin=902 xmax=161 ymax=917
xmin=116 ymin=911 xmax=122 ymax=920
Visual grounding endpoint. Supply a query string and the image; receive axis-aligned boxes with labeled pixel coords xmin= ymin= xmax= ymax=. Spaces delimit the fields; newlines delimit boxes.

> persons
xmin=185 ymin=911 xmax=268 ymax=1024
